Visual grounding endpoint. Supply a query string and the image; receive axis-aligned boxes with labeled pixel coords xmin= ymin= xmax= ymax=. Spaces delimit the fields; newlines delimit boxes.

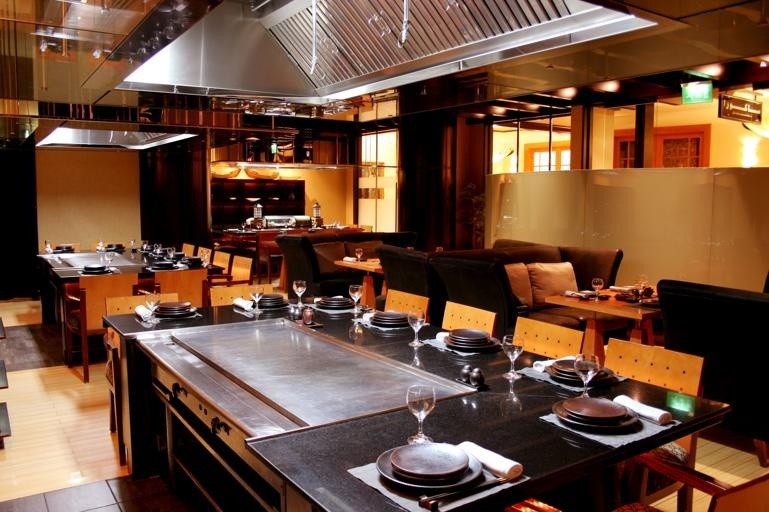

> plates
xmin=153 ymin=262 xmax=173 ymax=269
xmin=154 ymin=302 xmax=197 ymax=317
xmin=545 ymin=360 xmax=614 ymax=384
xmin=82 ymin=264 xmax=110 ymax=274
xmin=368 ymin=311 xmax=416 ymax=324
xmin=445 ymin=329 xmax=499 ymax=351
xmin=378 ymin=443 xmax=484 ymax=488
xmin=552 ymin=397 xmax=639 ymax=431
xmin=315 ymin=298 xmax=355 ymax=308
xmin=252 ymin=294 xmax=289 ymax=308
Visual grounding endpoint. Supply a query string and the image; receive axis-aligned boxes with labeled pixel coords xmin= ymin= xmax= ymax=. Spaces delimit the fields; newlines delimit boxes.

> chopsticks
xmin=420 ymin=476 xmax=507 ymax=505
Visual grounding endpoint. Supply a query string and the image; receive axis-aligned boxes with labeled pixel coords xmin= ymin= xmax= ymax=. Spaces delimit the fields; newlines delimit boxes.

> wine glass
xmin=145 ymin=293 xmax=160 ymax=324
xmin=127 ymin=239 xmax=176 ymax=262
xmin=503 ymin=335 xmax=524 ymax=380
xmin=349 ymin=285 xmax=363 ymax=320
xmin=249 ymin=285 xmax=263 ymax=315
xmin=201 ymin=248 xmax=210 ymax=267
xmin=407 ymin=386 xmax=437 ymax=444
xmin=633 ymin=275 xmax=647 ymax=303
xmin=573 ymin=353 xmax=599 ymax=397
xmin=356 ymin=248 xmax=362 ymax=263
xmin=408 ymin=308 xmax=426 ymax=347
xmin=293 ymin=280 xmax=306 ymax=309
xmin=592 ymin=277 xmax=604 ymax=303
xmin=104 ymin=252 xmax=115 ymax=272
